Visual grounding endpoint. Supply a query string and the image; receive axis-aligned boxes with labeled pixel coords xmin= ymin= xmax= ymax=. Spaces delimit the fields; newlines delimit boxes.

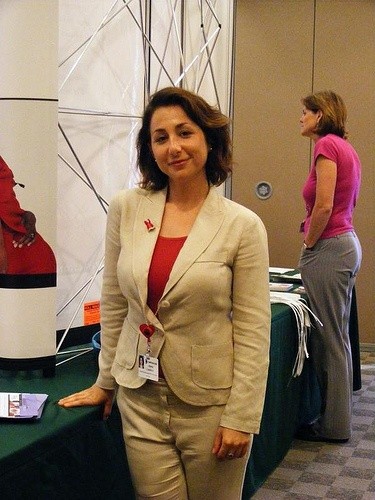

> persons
xmin=298 ymin=90 xmax=362 ymax=444
xmin=138 ymin=355 xmax=145 ymax=369
xmin=58 ymin=86 xmax=271 ymax=500
xmin=0 ymin=155 xmax=56 ymax=275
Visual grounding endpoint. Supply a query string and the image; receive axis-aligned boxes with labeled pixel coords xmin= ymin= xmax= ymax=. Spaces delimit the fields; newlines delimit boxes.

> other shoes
xmin=302 ymin=425 xmax=350 ymax=443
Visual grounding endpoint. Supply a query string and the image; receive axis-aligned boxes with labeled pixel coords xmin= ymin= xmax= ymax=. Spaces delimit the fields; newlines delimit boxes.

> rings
xmin=30 ymin=233 xmax=34 ymax=238
xmin=226 ymin=453 xmax=235 ymax=458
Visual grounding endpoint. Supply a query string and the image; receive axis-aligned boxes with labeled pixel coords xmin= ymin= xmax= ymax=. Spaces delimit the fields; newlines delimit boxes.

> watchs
xmin=303 ymin=240 xmax=313 ymax=251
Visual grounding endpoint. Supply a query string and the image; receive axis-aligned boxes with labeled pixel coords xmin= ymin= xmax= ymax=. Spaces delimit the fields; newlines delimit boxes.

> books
xmin=269 ymin=282 xmax=293 ymax=292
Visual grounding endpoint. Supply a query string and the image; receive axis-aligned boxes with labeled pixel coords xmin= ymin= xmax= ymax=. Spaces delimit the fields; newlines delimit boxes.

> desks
xmin=0 ymin=269 xmax=362 ymax=500
